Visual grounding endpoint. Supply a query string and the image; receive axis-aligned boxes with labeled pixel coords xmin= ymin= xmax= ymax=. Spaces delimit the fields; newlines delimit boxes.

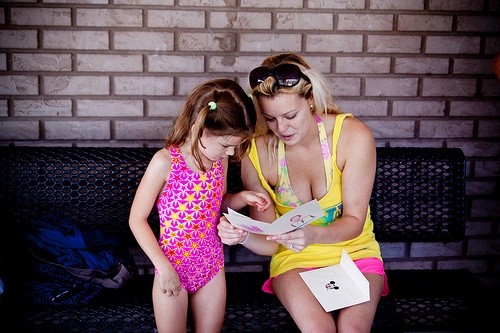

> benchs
xmin=0 ymin=136 xmax=500 ymax=333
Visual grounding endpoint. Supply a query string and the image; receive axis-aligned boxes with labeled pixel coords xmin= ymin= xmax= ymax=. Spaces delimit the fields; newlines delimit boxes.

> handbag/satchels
xmin=24 ymin=229 xmax=144 ymax=306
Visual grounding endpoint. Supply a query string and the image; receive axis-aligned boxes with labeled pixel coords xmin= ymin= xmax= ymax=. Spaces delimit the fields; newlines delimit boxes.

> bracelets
xmin=239 ymin=232 xmax=250 ymax=244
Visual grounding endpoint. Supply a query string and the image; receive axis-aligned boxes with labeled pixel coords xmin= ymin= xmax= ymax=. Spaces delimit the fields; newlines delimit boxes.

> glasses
xmin=249 ymin=64 xmax=311 ymax=90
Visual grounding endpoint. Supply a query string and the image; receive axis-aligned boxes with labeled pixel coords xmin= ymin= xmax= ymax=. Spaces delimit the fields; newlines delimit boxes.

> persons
xmin=128 ymin=79 xmax=273 ymax=333
xmin=290 ymin=214 xmax=315 ymax=228
xmin=217 ymin=53 xmax=390 ymax=333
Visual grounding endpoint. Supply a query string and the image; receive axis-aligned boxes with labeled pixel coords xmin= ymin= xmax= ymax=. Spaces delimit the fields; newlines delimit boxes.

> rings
xmin=289 ymin=244 xmax=295 ymax=248
